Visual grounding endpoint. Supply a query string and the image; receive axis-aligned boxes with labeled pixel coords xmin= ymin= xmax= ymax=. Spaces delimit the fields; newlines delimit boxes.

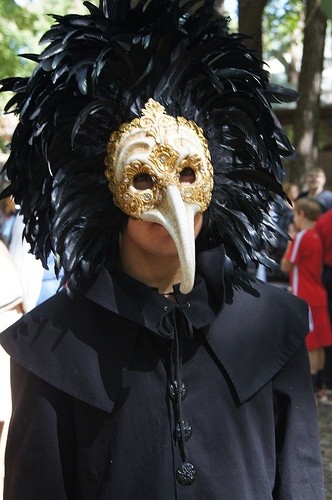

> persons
xmin=281 ymin=198 xmax=328 ymax=407
xmin=0 ymin=1 xmax=323 ymax=500
xmin=285 ymin=182 xmax=301 ymax=214
xmin=0 ymin=163 xmax=75 ymax=445
xmin=315 ymin=205 xmax=332 ymax=328
xmin=294 ymin=167 xmax=331 ymax=212
